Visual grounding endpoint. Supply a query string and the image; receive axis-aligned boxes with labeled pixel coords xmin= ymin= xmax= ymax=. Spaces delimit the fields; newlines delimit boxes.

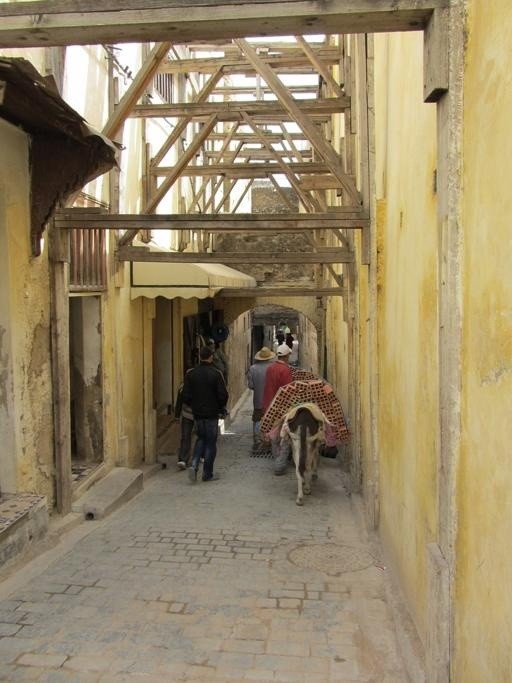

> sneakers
xmin=177 ymin=460 xmax=187 ymax=471
xmin=188 ymin=466 xmax=197 ymax=482
xmin=206 ymin=475 xmax=220 ymax=482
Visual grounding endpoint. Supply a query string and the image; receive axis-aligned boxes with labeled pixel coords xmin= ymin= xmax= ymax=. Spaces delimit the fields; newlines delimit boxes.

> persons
xmin=173 ymin=330 xmax=295 ymax=482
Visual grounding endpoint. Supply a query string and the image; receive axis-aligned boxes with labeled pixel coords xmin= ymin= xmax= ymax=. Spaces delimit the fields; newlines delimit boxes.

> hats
xmin=255 ymin=347 xmax=275 ymax=360
xmin=276 ymin=344 xmax=293 ymax=357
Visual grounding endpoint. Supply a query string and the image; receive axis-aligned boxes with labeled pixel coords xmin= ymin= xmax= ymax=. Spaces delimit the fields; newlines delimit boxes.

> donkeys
xmin=286 ymin=366 xmax=325 ymax=506
